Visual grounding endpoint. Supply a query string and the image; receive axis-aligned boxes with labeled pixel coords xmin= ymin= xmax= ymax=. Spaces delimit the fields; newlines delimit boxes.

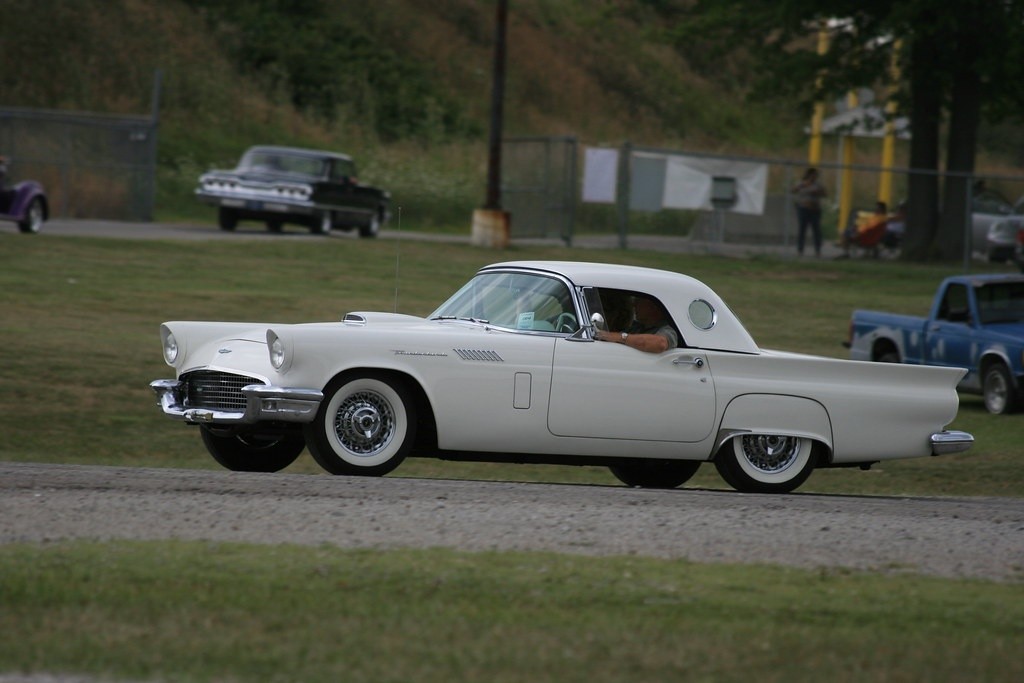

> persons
xmin=834 ymin=203 xmax=887 ymax=259
xmin=792 ymin=168 xmax=826 ymax=258
xmin=598 ymin=295 xmax=678 ymax=353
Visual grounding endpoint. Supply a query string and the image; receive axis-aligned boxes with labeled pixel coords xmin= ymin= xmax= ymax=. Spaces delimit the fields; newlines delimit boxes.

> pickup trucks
xmin=842 ymin=273 xmax=1024 ymax=414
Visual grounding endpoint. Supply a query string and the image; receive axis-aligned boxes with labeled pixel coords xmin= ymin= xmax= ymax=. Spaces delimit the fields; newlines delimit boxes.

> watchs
xmin=622 ymin=332 xmax=628 ymax=343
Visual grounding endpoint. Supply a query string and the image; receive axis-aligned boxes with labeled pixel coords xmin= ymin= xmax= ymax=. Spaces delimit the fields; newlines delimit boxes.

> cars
xmin=194 ymin=145 xmax=391 ymax=237
xmin=877 ymin=193 xmax=1024 ymax=269
xmin=146 ymin=260 xmax=978 ymax=495
xmin=0 ymin=160 xmax=50 ymax=233
